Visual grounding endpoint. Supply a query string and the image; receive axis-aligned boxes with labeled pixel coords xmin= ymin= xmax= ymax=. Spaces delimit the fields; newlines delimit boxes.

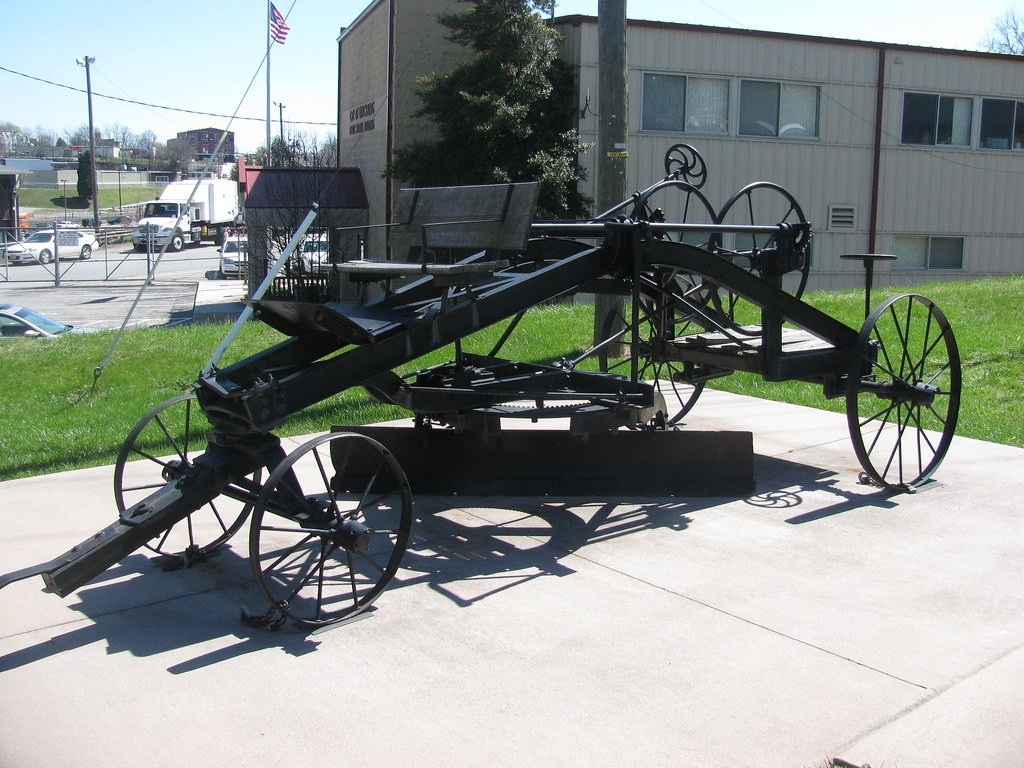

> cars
xmin=300 ymin=232 xmax=333 ymax=273
xmin=56 ymin=220 xmax=80 ymax=229
xmin=2 ymin=228 xmax=100 ymax=265
xmin=108 ymin=215 xmax=132 ymax=226
xmin=216 ymin=236 xmax=249 ymax=279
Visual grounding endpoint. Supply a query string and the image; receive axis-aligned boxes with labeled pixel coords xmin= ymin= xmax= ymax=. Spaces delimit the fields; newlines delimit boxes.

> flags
xmin=268 ymin=0 xmax=290 ymax=45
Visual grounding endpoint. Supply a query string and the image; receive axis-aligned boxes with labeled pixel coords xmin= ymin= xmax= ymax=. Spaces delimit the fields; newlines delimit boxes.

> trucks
xmin=133 ymin=178 xmax=239 ymax=252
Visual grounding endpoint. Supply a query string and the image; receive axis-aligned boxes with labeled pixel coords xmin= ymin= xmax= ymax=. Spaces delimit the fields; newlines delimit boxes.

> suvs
xmin=82 ymin=219 xmax=102 ymax=228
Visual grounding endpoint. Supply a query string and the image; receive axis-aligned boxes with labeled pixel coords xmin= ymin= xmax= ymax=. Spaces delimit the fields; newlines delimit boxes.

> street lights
xmin=274 ymin=100 xmax=288 ymax=167
xmin=61 ymin=179 xmax=68 ymax=221
